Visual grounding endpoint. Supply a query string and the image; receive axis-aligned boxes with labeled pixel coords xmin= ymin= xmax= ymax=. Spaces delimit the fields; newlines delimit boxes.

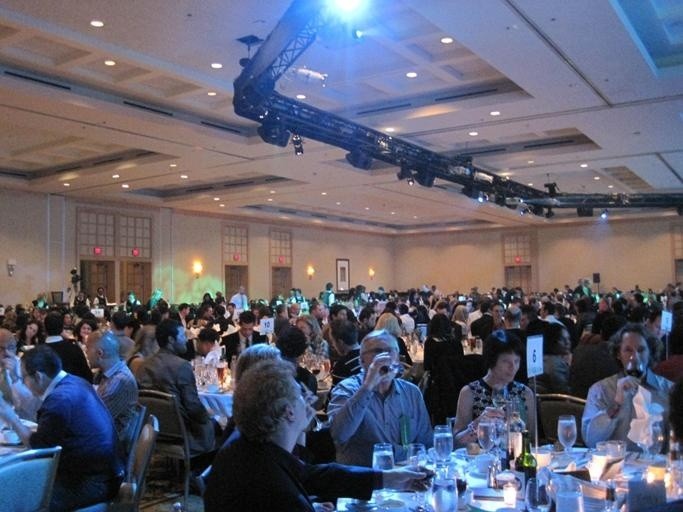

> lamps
xmin=192 ymin=260 xmax=203 ymax=279
xmin=306 ymin=265 xmax=314 ymax=280
xmin=600 ymin=208 xmax=611 ymax=219
xmin=520 ymin=206 xmax=534 ymax=215
xmin=292 ymin=135 xmax=303 ymax=157
xmin=478 ymin=192 xmax=484 ymax=203
xmin=369 ymin=269 xmax=375 ymax=280
xmin=404 ymin=168 xmax=414 ymax=186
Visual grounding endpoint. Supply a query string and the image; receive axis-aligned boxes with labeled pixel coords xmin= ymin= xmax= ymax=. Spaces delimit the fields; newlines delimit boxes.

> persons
xmin=646 ymin=382 xmax=683 ymax=512
xmin=223 ymin=342 xmax=336 ymax=463
xmin=581 ymin=324 xmax=676 ymax=454
xmin=1 ymin=280 xmax=683 ymax=425
xmin=0 ymin=343 xmax=127 ymax=512
xmin=327 ymin=328 xmax=434 ymax=467
xmin=453 ymin=328 xmax=535 ymax=444
xmin=134 ymin=317 xmax=220 ymax=497
xmin=86 ymin=331 xmax=138 ymax=455
xmin=203 ymin=359 xmax=435 ymax=512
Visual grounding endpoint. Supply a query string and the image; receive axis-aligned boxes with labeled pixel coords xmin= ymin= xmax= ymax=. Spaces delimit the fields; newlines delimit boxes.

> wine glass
xmin=381 ymin=352 xmax=404 ymax=380
xmin=194 ymin=355 xmax=236 ymax=392
xmin=626 ymin=360 xmax=644 ymax=395
xmin=491 ymin=384 xmax=509 ymax=424
xmin=304 ymin=354 xmax=331 ymax=381
xmin=372 ymin=414 xmax=664 ymax=512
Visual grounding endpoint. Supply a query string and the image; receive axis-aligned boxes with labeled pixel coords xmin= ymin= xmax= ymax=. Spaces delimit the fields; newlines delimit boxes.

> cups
xmin=2 ymin=430 xmax=18 ymax=442
xmin=469 ymin=337 xmax=483 ymax=352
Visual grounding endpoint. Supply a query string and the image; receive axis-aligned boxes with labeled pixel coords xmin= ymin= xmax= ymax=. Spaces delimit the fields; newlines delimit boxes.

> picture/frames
xmin=336 ymin=258 xmax=350 ymax=292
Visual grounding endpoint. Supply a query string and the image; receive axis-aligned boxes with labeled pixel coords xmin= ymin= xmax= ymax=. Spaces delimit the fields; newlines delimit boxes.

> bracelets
xmin=468 ymin=421 xmax=478 ymax=441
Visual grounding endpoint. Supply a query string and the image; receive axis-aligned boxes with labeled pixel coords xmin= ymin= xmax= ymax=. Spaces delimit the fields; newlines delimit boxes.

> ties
xmin=246 ymin=337 xmax=249 ymax=348
